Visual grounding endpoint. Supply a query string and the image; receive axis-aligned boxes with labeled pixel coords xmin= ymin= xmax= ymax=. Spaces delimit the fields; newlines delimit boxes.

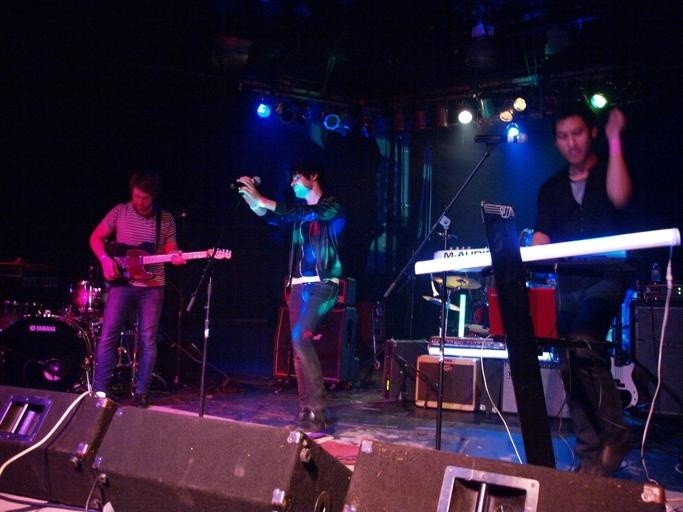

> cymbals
xmin=435 ymin=276 xmax=480 ymax=289
xmin=422 ymin=295 xmax=461 ymax=312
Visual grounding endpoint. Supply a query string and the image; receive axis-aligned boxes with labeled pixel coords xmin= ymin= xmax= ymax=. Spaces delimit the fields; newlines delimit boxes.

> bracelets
xmin=98 ymin=253 xmax=108 ymax=263
xmin=250 ymin=204 xmax=260 ymax=211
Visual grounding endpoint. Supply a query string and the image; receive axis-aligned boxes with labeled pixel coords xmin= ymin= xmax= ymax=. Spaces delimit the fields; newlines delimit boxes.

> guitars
xmin=609 ymin=306 xmax=638 ymax=411
xmin=105 ymin=242 xmax=231 ymax=280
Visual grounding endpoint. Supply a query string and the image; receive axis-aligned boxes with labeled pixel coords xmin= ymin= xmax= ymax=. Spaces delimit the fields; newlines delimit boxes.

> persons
xmin=87 ymin=171 xmax=187 ymax=408
xmin=235 ymin=164 xmax=346 ymax=439
xmin=527 ymin=97 xmax=633 ymax=476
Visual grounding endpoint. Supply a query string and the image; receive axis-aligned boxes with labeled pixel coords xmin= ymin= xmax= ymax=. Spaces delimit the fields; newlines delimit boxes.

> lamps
xmin=390 ymin=84 xmax=539 ymax=141
xmin=254 ymin=91 xmax=380 ymax=141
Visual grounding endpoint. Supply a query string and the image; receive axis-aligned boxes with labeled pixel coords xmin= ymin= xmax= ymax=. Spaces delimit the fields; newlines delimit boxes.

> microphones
xmin=229 ymin=177 xmax=262 ymax=191
xmin=474 ymin=133 xmax=529 ymax=146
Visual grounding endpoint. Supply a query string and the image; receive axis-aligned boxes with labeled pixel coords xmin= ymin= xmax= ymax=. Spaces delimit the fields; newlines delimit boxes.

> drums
xmin=465 ymin=303 xmax=489 ymax=333
xmin=0 ymin=315 xmax=93 ymax=392
xmin=70 ymin=280 xmax=102 ymax=313
xmin=4 ymin=300 xmax=43 ymax=314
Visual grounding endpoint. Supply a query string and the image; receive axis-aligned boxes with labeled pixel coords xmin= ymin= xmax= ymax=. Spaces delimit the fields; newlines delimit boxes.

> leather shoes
xmin=283 ymin=404 xmax=332 ymax=434
xmin=126 ymin=393 xmax=148 ymax=407
xmin=577 ymin=445 xmax=622 ymax=477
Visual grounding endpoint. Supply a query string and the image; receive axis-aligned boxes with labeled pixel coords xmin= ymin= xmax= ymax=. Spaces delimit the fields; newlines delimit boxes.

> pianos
xmin=433 ymin=246 xmax=626 ymax=262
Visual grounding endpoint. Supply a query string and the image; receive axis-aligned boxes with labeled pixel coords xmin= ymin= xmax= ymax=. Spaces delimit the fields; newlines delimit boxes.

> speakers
xmin=0 ymin=384 xmax=115 ymax=510
xmin=94 ymin=406 xmax=350 ymax=511
xmin=340 ymin=439 xmax=667 ymax=512
xmin=631 ymin=303 xmax=682 ymax=416
xmin=274 ymin=306 xmax=357 ymax=385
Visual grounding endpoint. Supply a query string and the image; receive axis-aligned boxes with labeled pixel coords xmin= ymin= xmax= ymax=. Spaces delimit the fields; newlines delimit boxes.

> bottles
xmin=651 ymin=262 xmax=661 ymax=282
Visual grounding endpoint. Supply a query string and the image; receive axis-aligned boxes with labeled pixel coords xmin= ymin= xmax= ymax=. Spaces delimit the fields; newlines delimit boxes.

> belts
xmin=290 ymin=274 xmax=341 ymax=285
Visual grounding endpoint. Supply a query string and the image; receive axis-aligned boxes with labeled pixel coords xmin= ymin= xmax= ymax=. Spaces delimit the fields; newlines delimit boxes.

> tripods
xmin=86 ymin=314 xmax=171 ymax=395
